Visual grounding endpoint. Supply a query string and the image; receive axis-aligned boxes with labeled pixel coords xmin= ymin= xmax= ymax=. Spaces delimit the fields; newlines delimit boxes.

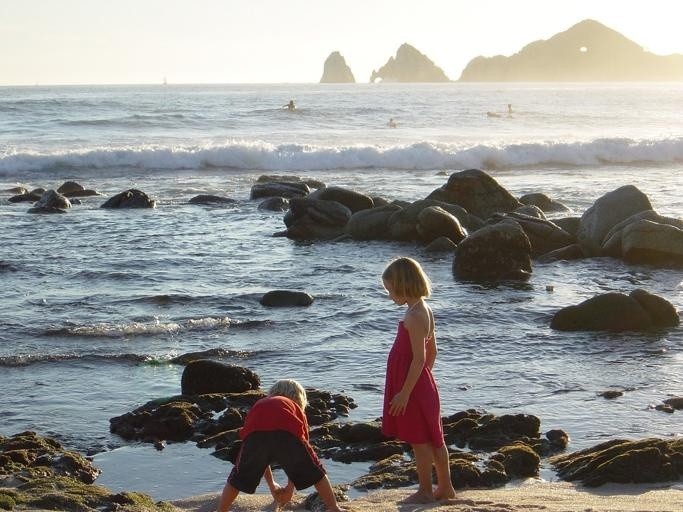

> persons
xmin=507 ymin=102 xmax=513 ymax=112
xmin=282 ymin=100 xmax=296 ymax=112
xmin=216 ymin=377 xmax=349 ymax=512
xmin=487 ymin=111 xmax=501 ymax=119
xmin=381 ymin=257 xmax=458 ymax=506
xmin=387 ymin=117 xmax=397 ymax=127
